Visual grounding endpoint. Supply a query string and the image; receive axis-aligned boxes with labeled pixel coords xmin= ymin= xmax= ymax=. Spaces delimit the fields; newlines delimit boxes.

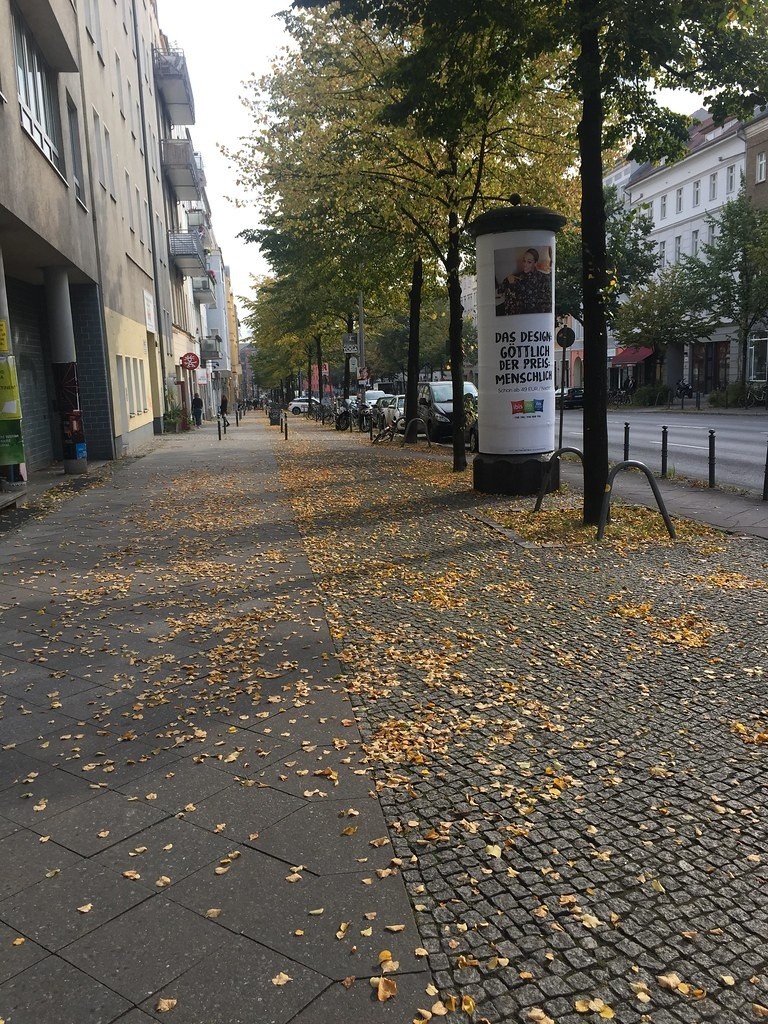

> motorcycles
xmin=675 ymin=378 xmax=694 ymax=399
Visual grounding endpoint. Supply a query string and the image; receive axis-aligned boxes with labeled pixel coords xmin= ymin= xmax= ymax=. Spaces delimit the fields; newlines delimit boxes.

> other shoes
xmin=226 ymin=424 xmax=230 ymax=426
xmin=222 ymin=425 xmax=225 ymax=427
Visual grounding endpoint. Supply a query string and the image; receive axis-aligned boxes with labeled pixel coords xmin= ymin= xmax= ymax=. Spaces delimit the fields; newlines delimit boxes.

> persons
xmin=192 ymin=393 xmax=203 ymax=429
xmin=624 ymin=376 xmax=636 ymax=405
xmin=498 ymin=249 xmax=552 ymax=315
xmin=241 ymin=399 xmax=257 ymax=411
xmin=220 ymin=395 xmax=230 ymax=427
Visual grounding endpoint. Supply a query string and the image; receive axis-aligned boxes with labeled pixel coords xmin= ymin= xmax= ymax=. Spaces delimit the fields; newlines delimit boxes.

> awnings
xmin=612 ymin=345 xmax=653 ymax=362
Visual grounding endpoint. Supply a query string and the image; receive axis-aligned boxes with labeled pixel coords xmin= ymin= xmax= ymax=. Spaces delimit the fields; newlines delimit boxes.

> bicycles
xmin=737 ymin=381 xmax=768 ymax=409
xmin=372 ymin=418 xmax=400 ymax=445
xmin=607 ymin=387 xmax=633 ymax=408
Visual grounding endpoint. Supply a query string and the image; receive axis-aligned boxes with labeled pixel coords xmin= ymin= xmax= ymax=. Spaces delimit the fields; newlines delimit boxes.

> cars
xmin=555 ymin=387 xmax=584 ymax=409
xmin=305 ymin=389 xmax=405 ymax=434
xmin=464 ymin=415 xmax=479 ymax=453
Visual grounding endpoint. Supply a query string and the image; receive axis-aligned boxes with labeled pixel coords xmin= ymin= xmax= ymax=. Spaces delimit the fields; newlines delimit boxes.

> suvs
xmin=404 ymin=380 xmax=479 ymax=444
xmin=287 ymin=397 xmax=324 ymax=415
